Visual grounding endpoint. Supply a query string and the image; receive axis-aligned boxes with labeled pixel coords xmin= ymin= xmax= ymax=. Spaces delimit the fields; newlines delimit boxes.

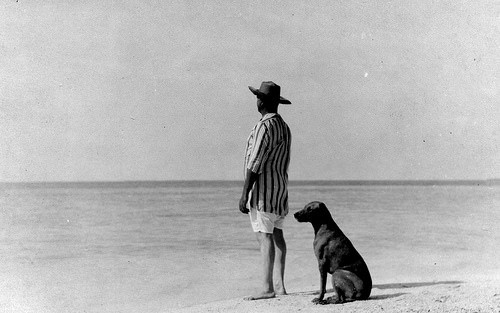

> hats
xmin=248 ymin=80 xmax=292 ymax=106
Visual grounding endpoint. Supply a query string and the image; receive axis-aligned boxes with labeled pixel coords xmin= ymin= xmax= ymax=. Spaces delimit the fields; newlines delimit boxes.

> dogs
xmin=294 ymin=201 xmax=372 ymax=304
